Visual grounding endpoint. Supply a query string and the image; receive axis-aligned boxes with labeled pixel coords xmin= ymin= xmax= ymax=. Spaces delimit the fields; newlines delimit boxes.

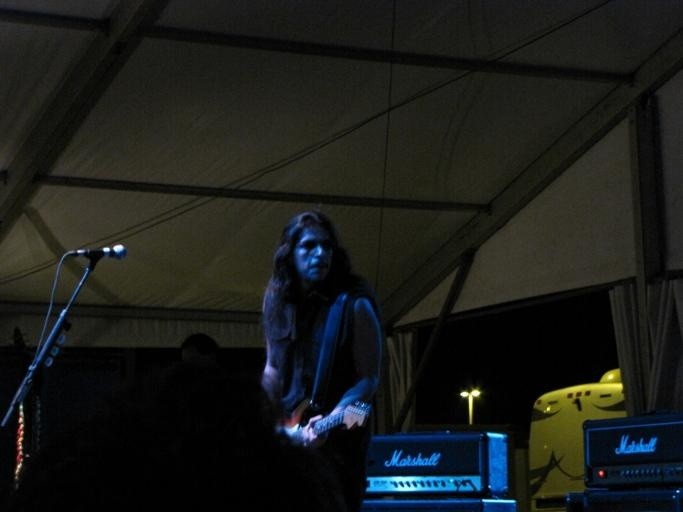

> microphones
xmin=66 ymin=244 xmax=128 ymax=260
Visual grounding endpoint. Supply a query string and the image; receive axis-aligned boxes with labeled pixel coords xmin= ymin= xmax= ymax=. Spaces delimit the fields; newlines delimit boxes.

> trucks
xmin=527 ymin=366 xmax=626 ymax=512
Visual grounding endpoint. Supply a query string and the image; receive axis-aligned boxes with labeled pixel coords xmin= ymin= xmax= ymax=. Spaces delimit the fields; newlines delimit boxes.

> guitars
xmin=273 ymin=400 xmax=370 ymax=452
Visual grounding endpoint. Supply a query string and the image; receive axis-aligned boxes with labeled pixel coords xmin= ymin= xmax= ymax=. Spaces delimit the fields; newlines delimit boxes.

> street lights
xmin=457 ymin=387 xmax=482 ymax=426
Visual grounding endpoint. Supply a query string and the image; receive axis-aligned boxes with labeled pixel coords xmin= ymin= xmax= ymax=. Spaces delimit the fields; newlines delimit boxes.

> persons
xmin=259 ymin=210 xmax=382 ymax=507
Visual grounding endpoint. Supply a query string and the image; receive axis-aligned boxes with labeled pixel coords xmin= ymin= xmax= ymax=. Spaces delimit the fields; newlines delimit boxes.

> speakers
xmin=366 ymin=430 xmax=510 ymax=495
xmin=582 ymin=413 xmax=682 ymax=486
